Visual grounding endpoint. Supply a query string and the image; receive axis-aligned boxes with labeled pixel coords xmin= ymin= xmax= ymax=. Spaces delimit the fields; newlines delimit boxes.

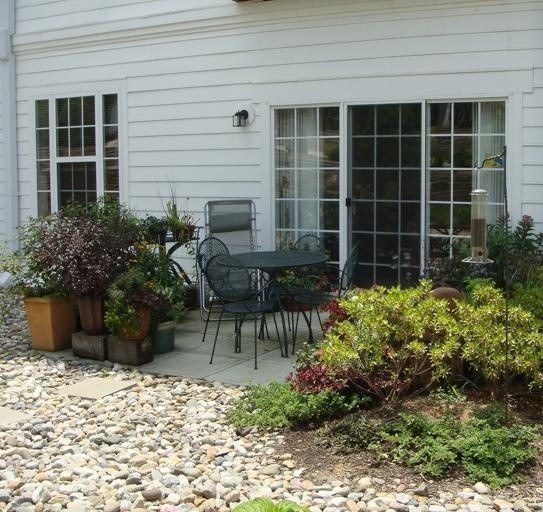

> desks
xmin=211 ymin=247 xmax=330 ymax=354
xmin=138 ymin=236 xmax=201 ymax=287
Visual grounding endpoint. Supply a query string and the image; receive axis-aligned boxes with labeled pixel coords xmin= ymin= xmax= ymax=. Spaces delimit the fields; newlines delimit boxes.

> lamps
xmin=230 ymin=107 xmax=248 ymax=128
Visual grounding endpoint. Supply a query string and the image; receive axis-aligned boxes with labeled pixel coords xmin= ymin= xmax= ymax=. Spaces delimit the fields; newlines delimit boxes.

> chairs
xmin=193 ymin=235 xmax=262 ymax=346
xmin=253 ymin=233 xmax=329 ymax=337
xmin=202 ymin=252 xmax=287 ymax=369
xmin=287 ymin=238 xmax=364 ymax=356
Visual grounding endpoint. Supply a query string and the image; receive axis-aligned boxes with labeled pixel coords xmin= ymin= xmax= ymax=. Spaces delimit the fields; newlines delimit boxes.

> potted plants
xmin=0 ymin=240 xmax=76 ymax=353
xmin=136 ymin=210 xmax=171 ymax=240
xmin=102 ymin=266 xmax=172 ymax=338
xmin=160 ymin=173 xmax=203 ymax=237
xmin=31 ymin=210 xmax=133 ymax=335
